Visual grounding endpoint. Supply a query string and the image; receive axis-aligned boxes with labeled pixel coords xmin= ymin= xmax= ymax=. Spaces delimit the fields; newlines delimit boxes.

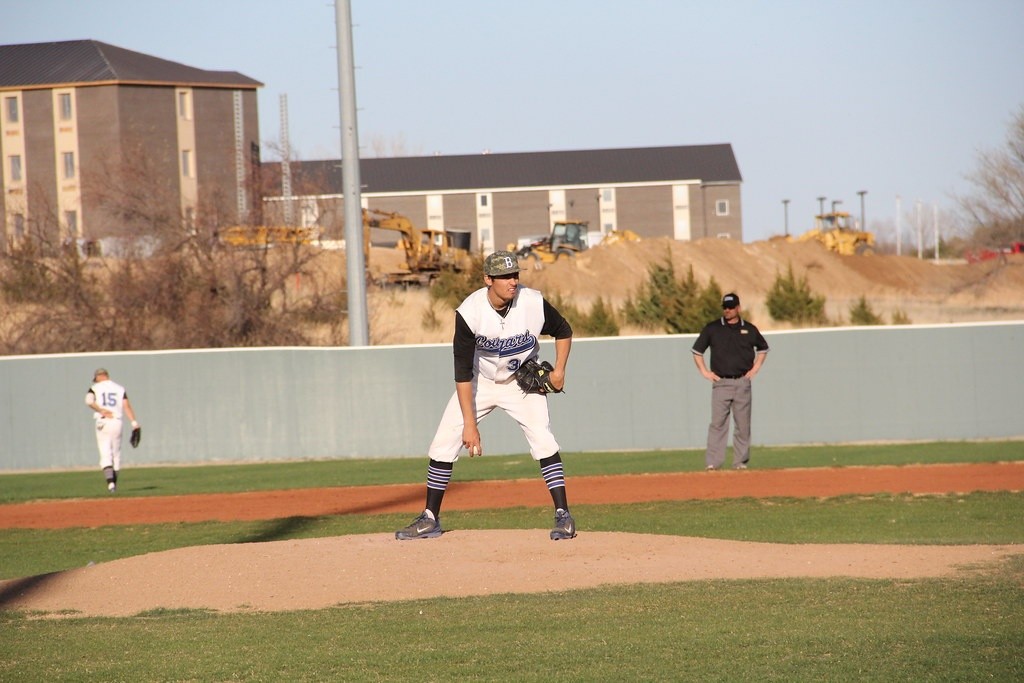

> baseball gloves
xmin=513 ymin=358 xmax=563 ymax=394
xmin=130 ymin=426 xmax=142 ymax=447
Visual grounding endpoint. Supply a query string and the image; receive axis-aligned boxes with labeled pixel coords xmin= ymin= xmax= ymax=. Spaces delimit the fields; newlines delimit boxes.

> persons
xmin=395 ymin=250 xmax=575 ymax=541
xmin=691 ymin=293 xmax=769 ymax=472
xmin=85 ymin=368 xmax=140 ymax=493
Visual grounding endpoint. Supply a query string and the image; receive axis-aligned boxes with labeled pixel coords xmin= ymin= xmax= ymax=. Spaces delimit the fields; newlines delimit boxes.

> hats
xmin=721 ymin=293 xmax=739 ymax=307
xmin=92 ymin=368 xmax=110 ymax=382
xmin=484 ymin=250 xmax=528 ymax=276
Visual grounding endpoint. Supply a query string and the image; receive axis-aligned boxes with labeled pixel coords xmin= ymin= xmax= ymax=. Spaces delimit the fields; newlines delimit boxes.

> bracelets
xmin=131 ymin=420 xmax=137 ymax=426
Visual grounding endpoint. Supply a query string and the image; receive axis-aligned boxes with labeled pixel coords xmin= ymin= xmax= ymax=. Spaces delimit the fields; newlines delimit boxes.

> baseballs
xmin=469 ymin=446 xmax=479 ymax=455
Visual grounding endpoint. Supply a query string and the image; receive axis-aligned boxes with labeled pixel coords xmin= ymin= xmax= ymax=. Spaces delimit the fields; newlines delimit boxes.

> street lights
xmin=831 ymin=200 xmax=843 ymax=213
xmin=782 ymin=199 xmax=791 ymax=236
xmin=934 ymin=199 xmax=939 ymax=261
xmin=917 ymin=197 xmax=924 ymax=260
xmin=857 ymin=190 xmax=868 ymax=232
xmin=896 ymin=194 xmax=901 ymax=257
xmin=817 ymin=197 xmax=827 ymax=215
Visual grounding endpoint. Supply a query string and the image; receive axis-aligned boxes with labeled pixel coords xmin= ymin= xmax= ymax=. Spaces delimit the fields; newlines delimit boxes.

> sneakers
xmin=395 ymin=510 xmax=442 ymax=540
xmin=549 ymin=509 xmax=577 ymax=540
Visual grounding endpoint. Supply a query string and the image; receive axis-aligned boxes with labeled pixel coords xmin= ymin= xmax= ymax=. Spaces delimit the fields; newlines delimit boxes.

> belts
xmin=720 ymin=374 xmax=745 ymax=379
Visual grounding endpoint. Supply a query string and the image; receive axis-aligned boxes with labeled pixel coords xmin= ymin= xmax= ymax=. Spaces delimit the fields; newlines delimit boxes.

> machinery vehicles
xmin=217 ymin=225 xmax=326 ymax=248
xmin=362 ymin=206 xmax=442 ymax=290
xmin=599 ymin=229 xmax=641 ymax=246
xmin=769 ymin=212 xmax=877 ymax=257
xmin=414 ymin=227 xmax=470 ymax=275
xmin=513 ymin=220 xmax=589 ymax=264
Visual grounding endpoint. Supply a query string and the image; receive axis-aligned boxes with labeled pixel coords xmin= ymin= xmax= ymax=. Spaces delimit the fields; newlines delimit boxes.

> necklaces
xmin=486 ymin=296 xmax=513 ymax=329
